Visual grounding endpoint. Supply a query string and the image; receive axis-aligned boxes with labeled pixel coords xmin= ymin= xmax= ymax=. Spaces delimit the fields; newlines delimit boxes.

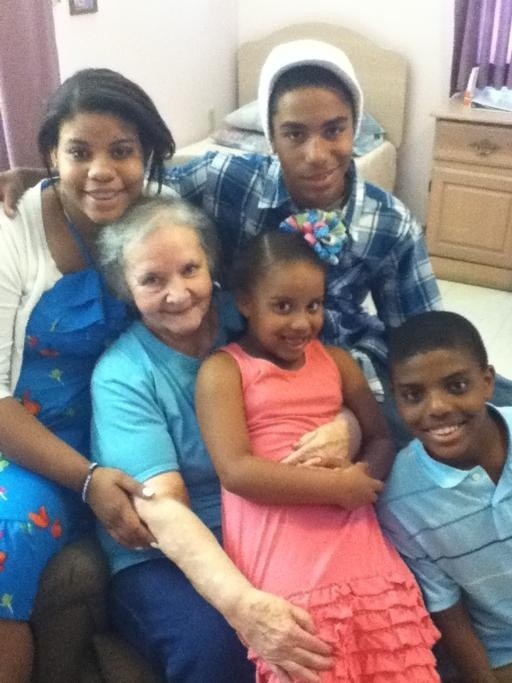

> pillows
xmin=224 ymin=100 xmax=389 ymax=135
xmin=213 ymin=129 xmax=383 ymax=158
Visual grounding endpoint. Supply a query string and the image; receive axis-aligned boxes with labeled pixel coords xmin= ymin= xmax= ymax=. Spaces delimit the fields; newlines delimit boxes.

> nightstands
xmin=427 ymin=93 xmax=512 ymax=290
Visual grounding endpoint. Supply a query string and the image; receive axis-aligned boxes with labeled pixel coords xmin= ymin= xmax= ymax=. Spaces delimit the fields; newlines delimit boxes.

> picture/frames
xmin=69 ymin=0 xmax=98 ymax=15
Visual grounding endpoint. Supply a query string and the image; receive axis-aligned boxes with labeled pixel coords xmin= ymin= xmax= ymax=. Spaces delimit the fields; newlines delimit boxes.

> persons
xmin=195 ymin=206 xmax=442 ymax=683
xmin=89 ymin=199 xmax=362 ymax=683
xmin=375 ymin=312 xmax=512 ymax=683
xmin=0 ymin=69 xmax=184 ymax=683
xmin=0 ymin=40 xmax=447 ymax=450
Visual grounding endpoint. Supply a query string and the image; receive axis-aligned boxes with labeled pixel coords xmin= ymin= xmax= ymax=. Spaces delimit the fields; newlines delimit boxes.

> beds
xmin=166 ymin=24 xmax=407 ymax=194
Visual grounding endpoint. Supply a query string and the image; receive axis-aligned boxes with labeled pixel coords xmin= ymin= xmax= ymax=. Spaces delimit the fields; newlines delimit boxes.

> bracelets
xmin=81 ymin=463 xmax=98 ymax=504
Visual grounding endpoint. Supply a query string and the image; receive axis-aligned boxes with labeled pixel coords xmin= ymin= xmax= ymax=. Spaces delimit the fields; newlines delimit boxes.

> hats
xmin=257 ymin=41 xmax=364 ymax=145
